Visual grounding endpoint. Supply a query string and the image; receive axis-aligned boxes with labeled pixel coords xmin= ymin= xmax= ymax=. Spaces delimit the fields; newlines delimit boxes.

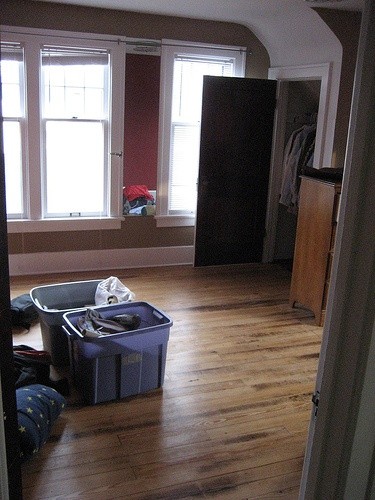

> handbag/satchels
xmin=10 ymin=293 xmax=39 ymax=330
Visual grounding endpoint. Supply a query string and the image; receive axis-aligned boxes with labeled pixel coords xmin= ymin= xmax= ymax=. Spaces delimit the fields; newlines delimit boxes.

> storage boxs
xmin=62 ymin=301 xmax=175 ymax=404
xmin=30 ymin=278 xmax=124 ymax=368
